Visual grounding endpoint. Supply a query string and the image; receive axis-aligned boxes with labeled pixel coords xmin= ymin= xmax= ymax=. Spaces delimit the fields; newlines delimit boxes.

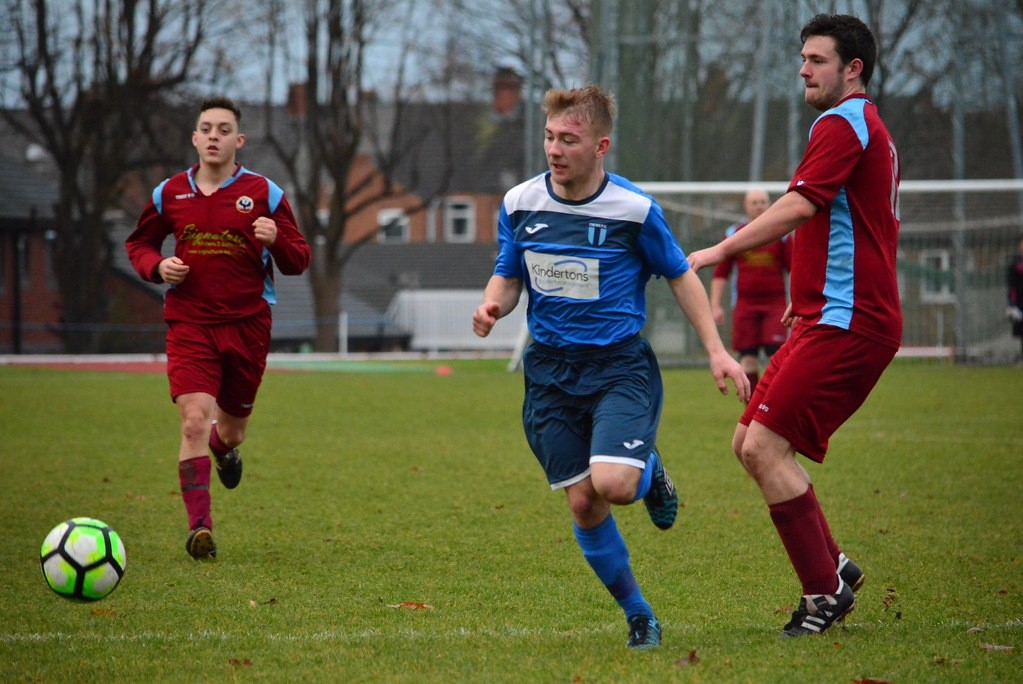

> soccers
xmin=40 ymin=517 xmax=126 ymax=602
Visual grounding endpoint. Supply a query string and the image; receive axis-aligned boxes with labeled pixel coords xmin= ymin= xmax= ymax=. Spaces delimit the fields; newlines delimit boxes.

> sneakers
xmin=626 ymin=616 xmax=661 ymax=649
xmin=643 ymin=445 xmax=678 ymax=530
xmin=186 ymin=526 xmax=216 ymax=559
xmin=209 ymin=420 xmax=243 ymax=489
xmin=837 ymin=551 xmax=866 ymax=591
xmin=783 ymin=573 xmax=857 ymax=637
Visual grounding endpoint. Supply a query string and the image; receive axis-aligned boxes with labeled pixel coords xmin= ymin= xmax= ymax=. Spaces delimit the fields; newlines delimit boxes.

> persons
xmin=472 ymin=87 xmax=751 ymax=648
xmin=710 ymin=187 xmax=791 ymax=406
xmin=684 ymin=9 xmax=903 ymax=638
xmin=124 ymin=96 xmax=314 ymax=566
